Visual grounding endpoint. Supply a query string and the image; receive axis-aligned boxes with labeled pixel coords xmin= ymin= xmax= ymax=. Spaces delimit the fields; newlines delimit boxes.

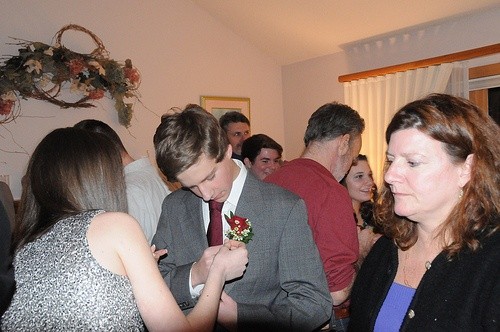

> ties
xmin=207 ymin=200 xmax=224 ymax=247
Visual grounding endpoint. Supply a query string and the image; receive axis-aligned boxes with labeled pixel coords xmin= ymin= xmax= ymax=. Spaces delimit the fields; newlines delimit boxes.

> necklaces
xmin=403 ymin=249 xmax=412 ymax=288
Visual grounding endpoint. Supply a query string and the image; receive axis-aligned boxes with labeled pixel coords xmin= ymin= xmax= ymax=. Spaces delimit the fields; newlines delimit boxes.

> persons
xmin=348 ymin=91 xmax=499 ymax=331
xmin=263 ymin=102 xmax=366 ymax=332
xmin=338 ymin=154 xmax=384 ymax=274
xmin=0 ymin=182 xmax=20 ymax=316
xmin=149 ymin=103 xmax=333 ymax=332
xmin=241 ymin=134 xmax=283 ymax=182
xmin=1 ymin=126 xmax=249 ymax=331
xmin=219 ymin=112 xmax=251 ymax=160
xmin=73 ymin=119 xmax=172 ymax=247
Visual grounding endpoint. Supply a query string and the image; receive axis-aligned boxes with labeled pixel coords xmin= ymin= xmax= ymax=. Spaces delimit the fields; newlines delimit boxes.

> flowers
xmin=0 ymin=42 xmax=141 ymax=116
xmin=223 ymin=210 xmax=255 ymax=244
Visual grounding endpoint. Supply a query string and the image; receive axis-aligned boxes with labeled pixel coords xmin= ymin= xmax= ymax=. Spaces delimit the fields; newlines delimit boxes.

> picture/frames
xmin=200 ymin=96 xmax=250 ymax=122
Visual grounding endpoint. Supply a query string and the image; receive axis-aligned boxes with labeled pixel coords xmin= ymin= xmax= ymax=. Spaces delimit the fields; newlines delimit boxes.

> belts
xmin=333 ymin=307 xmax=350 ymax=320
